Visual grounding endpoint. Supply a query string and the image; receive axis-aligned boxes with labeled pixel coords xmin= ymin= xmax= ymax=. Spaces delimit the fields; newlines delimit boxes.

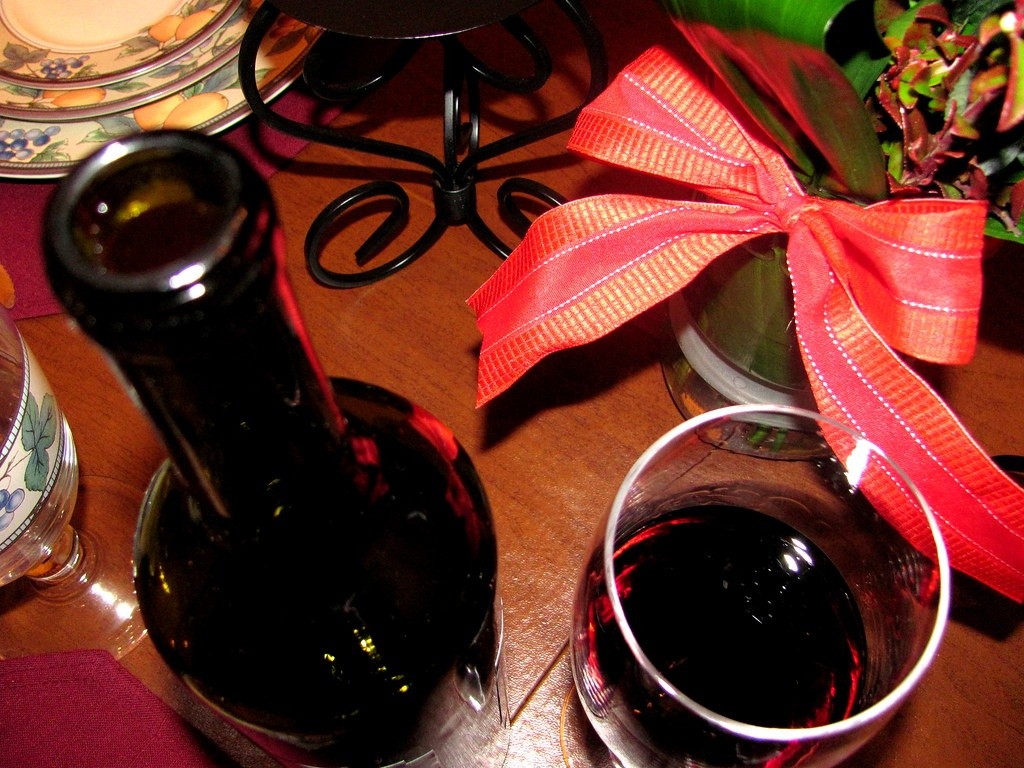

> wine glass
xmin=0 ymin=301 xmax=150 ymax=662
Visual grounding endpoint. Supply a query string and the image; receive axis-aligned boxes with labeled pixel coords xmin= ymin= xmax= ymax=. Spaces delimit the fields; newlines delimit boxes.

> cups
xmin=560 ymin=404 xmax=952 ymax=768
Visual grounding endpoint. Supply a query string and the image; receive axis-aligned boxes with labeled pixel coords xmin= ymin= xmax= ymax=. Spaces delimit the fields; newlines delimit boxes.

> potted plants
xmin=660 ymin=0 xmax=1024 ymax=461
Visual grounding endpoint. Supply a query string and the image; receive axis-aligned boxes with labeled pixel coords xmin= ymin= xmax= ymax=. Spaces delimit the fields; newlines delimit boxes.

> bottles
xmin=40 ymin=130 xmax=513 ymax=768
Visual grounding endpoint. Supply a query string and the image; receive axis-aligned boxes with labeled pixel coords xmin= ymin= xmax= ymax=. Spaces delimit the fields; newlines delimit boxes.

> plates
xmin=0 ymin=0 xmax=326 ymax=181
